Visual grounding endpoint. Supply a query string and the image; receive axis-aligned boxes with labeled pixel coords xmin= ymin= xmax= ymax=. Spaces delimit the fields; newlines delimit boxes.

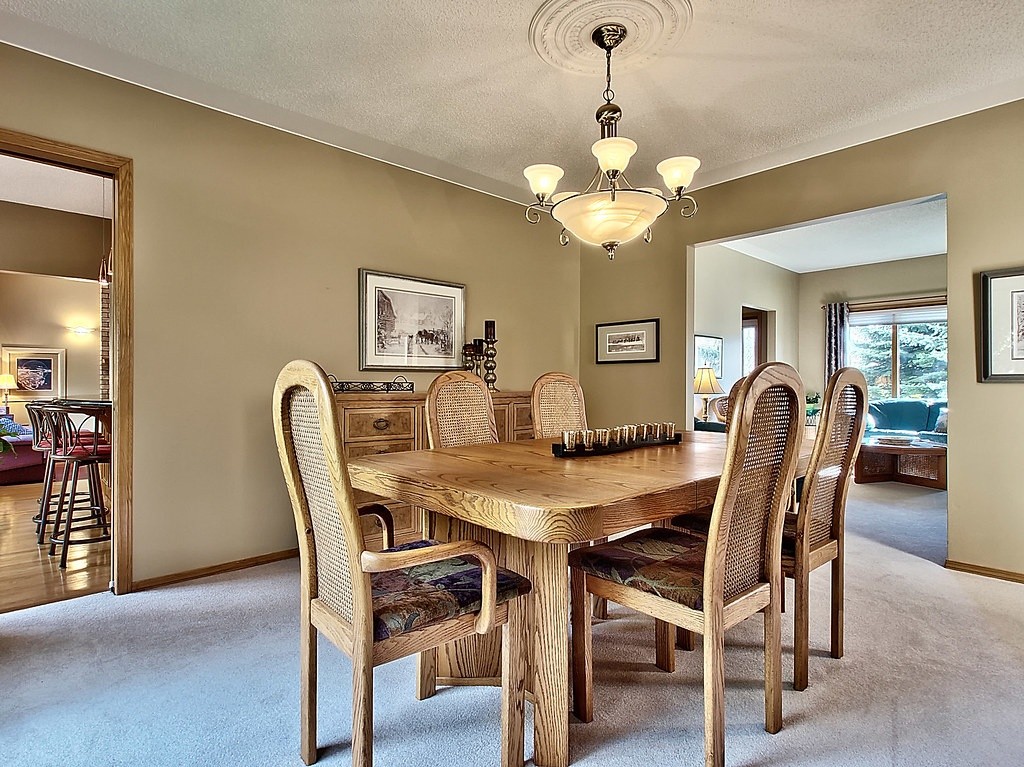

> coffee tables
xmin=854 ymin=436 xmax=948 ymax=491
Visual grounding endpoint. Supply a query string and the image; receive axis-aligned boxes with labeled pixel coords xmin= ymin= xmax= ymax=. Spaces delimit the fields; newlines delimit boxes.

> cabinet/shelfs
xmin=336 ymin=391 xmax=534 ymax=552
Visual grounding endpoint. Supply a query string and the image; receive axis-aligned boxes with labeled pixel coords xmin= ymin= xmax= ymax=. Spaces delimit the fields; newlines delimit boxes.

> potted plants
xmin=805 ymin=392 xmax=822 ymax=416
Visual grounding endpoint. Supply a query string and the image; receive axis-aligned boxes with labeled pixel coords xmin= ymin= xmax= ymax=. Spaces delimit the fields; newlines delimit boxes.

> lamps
xmin=107 ymin=180 xmax=114 ymax=276
xmin=522 ymin=22 xmax=701 ymax=262
xmin=98 ymin=177 xmax=109 ymax=286
xmin=0 ymin=374 xmax=19 ymax=406
xmin=693 ymin=368 xmax=727 ymax=422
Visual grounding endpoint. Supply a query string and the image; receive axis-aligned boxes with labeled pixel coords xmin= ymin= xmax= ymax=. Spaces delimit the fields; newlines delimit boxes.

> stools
xmin=24 ymin=400 xmax=112 ymax=571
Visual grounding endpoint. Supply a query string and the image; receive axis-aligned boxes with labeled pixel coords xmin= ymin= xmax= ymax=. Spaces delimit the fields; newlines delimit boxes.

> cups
xmin=615 ymin=426 xmax=628 ymax=442
xmin=661 ymin=422 xmax=676 ymax=439
xmin=624 ymin=424 xmax=637 ymax=440
xmin=648 ymin=423 xmax=661 ymax=440
xmin=580 ymin=430 xmax=595 ymax=448
xmin=636 ymin=423 xmax=648 ymax=440
xmin=562 ymin=430 xmax=578 ymax=450
xmin=609 ymin=429 xmax=619 ymax=444
xmin=595 ymin=428 xmax=610 ymax=446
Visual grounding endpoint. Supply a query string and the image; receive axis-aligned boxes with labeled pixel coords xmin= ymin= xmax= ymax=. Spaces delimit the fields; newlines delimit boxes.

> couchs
xmin=0 ymin=417 xmax=48 ymax=486
xmin=864 ymin=398 xmax=947 ymax=444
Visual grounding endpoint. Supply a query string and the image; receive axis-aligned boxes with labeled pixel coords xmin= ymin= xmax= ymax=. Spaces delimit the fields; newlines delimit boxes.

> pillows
xmin=0 ymin=418 xmax=25 ymax=435
xmin=932 ymin=408 xmax=947 ymax=433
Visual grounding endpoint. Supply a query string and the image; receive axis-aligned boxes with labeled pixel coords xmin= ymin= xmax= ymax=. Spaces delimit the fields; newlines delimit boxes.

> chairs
xmin=425 ymin=371 xmax=499 ymax=449
xmin=567 ymin=362 xmax=807 ymax=767
xmin=272 ymin=359 xmax=533 ymax=767
xmin=530 ymin=372 xmax=609 ymax=620
xmin=670 ymin=367 xmax=868 ymax=692
xmin=710 ymin=396 xmax=728 ymax=423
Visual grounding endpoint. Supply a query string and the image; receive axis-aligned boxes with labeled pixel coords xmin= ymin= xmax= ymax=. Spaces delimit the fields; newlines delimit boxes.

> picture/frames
xmin=0 ymin=344 xmax=67 ymax=403
xmin=596 ymin=317 xmax=660 ymax=364
xmin=358 ymin=267 xmax=467 ymax=372
xmin=980 ymin=266 xmax=1024 ymax=383
xmin=694 ymin=334 xmax=724 ymax=379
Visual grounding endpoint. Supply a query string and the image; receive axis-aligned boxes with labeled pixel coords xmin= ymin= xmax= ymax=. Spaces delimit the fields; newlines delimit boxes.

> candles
xmin=484 ymin=320 xmax=496 ymax=339
xmin=473 ymin=339 xmax=483 ymax=355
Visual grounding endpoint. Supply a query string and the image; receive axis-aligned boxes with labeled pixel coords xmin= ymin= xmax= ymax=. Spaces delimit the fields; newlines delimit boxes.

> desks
xmin=344 ymin=429 xmax=816 ymax=767
xmin=52 ymin=394 xmax=113 ymax=524
